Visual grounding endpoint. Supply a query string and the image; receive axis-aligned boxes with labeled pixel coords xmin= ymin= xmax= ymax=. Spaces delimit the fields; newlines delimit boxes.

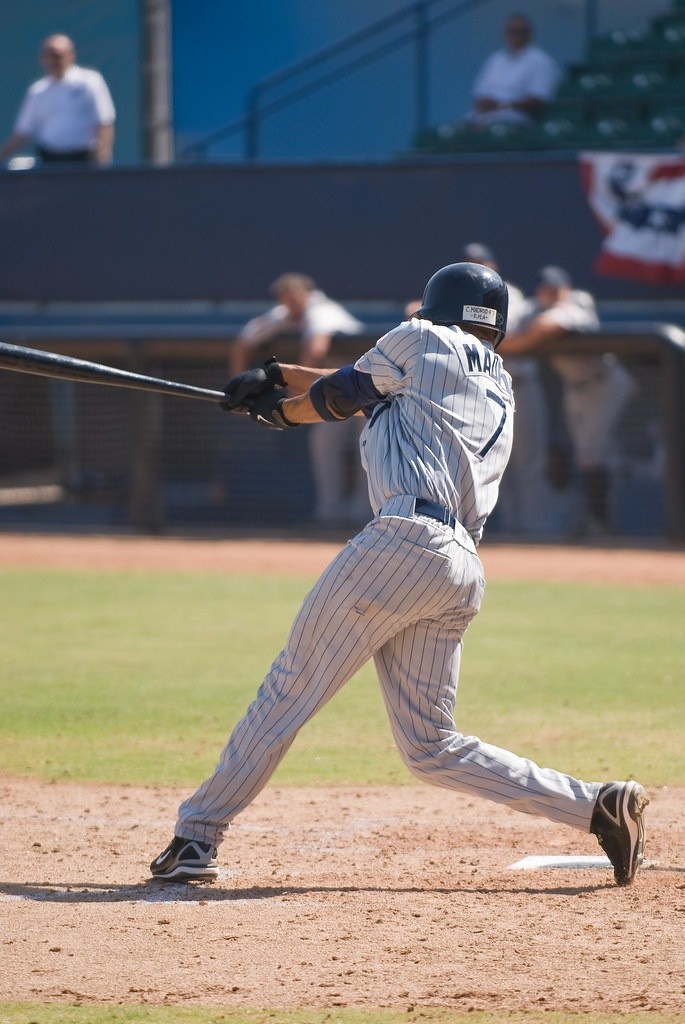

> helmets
xmin=409 ymin=261 xmax=509 ymax=350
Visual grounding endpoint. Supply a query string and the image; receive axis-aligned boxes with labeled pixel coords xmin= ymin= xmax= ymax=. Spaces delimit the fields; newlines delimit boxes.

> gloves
xmin=220 ymin=355 xmax=301 ymax=431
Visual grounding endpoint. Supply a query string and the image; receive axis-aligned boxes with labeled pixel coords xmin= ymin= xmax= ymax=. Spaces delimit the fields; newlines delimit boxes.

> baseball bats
xmin=1 ymin=341 xmax=227 ymax=401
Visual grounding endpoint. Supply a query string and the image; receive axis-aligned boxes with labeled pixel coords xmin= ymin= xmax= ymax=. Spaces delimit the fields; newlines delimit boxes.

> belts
xmin=378 ymin=497 xmax=455 ymax=529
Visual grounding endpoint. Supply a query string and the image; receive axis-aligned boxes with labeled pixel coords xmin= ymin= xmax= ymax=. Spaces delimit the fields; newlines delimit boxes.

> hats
xmin=533 ymin=263 xmax=575 ymax=289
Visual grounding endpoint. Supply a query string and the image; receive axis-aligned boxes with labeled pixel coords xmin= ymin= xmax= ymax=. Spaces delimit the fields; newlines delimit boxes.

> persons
xmin=148 ymin=263 xmax=651 ymax=888
xmin=405 ymin=243 xmax=637 ymax=539
xmin=230 ymin=274 xmax=368 ymax=523
xmin=0 ymin=34 xmax=116 ymax=170
xmin=461 ymin=14 xmax=560 ymax=124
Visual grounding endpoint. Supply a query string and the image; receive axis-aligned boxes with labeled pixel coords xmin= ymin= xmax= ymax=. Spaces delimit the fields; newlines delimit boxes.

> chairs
xmin=412 ymin=0 xmax=685 ymax=152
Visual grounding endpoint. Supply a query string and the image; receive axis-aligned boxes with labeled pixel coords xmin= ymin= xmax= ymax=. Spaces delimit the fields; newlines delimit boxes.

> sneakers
xmin=151 ymin=837 xmax=219 ymax=882
xmin=590 ymin=781 xmax=648 ymax=886
